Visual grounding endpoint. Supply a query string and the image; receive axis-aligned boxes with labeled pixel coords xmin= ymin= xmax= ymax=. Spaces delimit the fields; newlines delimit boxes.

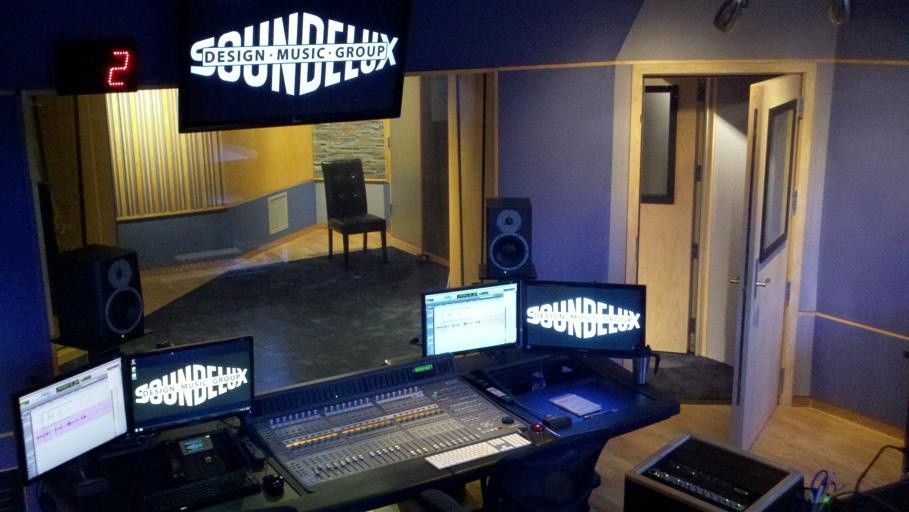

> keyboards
xmin=143 ymin=469 xmax=262 ymax=512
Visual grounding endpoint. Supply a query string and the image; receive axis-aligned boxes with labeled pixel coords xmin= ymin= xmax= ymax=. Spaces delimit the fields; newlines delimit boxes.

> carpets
xmin=57 ymin=242 xmax=450 ymax=394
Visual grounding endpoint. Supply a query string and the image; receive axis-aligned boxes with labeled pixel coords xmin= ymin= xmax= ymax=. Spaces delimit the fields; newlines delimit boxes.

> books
xmin=547 ymin=393 xmax=601 ymax=416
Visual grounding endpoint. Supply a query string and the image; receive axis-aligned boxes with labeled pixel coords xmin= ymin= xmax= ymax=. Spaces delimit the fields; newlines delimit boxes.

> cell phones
xmin=178 ymin=435 xmax=214 ymax=456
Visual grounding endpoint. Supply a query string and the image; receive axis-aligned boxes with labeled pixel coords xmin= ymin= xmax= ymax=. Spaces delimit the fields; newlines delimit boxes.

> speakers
xmin=485 ymin=196 xmax=532 ymax=272
xmin=54 ymin=244 xmax=143 ymax=346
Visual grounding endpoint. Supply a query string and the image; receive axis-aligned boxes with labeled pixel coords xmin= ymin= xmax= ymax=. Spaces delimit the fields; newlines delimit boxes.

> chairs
xmin=322 ymin=155 xmax=390 ymax=269
xmin=417 ymin=441 xmax=600 ymax=510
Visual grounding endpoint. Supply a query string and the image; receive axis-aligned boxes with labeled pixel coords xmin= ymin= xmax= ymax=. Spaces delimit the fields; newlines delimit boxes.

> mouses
xmin=263 ymin=472 xmax=283 ymax=491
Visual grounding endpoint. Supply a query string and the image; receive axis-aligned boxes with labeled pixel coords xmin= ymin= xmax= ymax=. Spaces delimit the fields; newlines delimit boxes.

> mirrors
xmin=638 ymin=82 xmax=677 ymax=203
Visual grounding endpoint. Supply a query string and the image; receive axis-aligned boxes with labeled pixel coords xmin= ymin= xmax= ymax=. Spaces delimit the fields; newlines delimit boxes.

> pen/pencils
xmin=811 ymin=476 xmax=831 ymax=512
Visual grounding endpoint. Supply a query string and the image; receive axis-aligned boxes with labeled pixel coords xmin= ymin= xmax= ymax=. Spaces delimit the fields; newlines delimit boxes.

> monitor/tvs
xmin=421 ymin=281 xmax=520 ymax=358
xmin=522 ymin=279 xmax=646 ymax=373
xmin=12 ymin=353 xmax=128 ymax=487
xmin=124 ymin=335 xmax=255 ymax=437
xmin=175 ymin=0 xmax=411 ymax=133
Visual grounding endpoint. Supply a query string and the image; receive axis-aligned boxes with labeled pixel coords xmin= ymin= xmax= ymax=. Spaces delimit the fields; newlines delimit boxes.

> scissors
xmin=810 ymin=470 xmax=837 ymax=503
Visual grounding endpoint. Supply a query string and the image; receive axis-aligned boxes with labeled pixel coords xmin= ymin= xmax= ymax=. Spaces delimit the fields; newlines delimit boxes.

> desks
xmin=0 ymin=339 xmax=683 ymax=511
